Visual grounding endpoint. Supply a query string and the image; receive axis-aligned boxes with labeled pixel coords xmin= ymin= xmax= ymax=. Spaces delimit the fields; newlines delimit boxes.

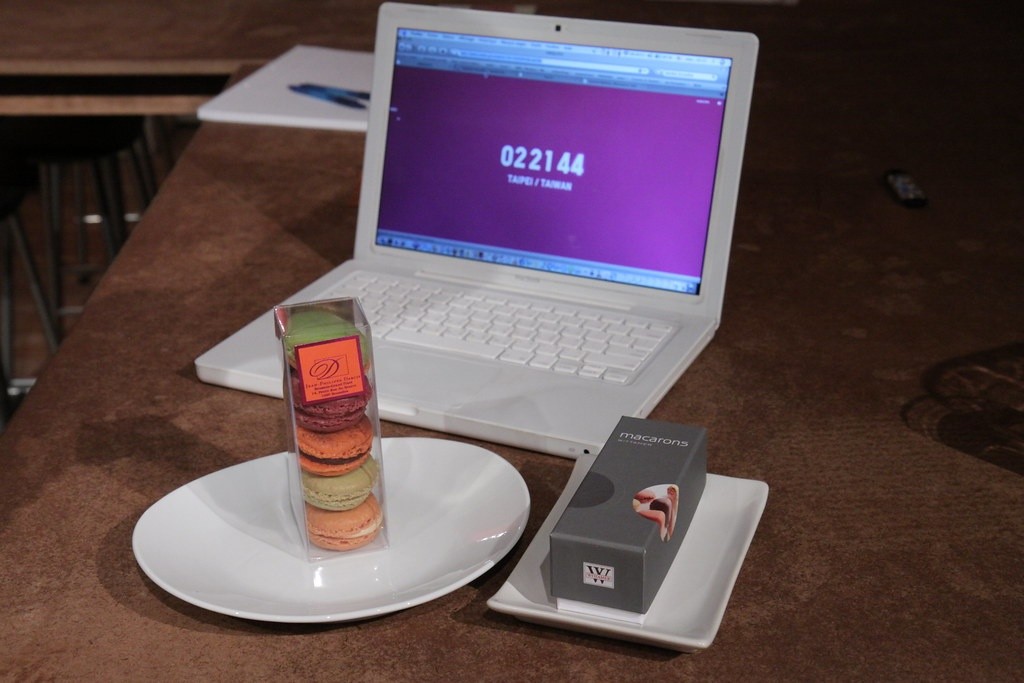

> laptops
xmin=195 ymin=2 xmax=759 ymax=459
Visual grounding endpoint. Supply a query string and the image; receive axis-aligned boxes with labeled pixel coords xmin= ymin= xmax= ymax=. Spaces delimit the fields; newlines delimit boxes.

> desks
xmin=0 ymin=55 xmax=1024 ymax=683
xmin=1 ymin=1 xmax=1021 ymax=116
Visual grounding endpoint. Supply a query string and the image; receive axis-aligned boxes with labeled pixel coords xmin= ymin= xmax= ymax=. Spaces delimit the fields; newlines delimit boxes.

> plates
xmin=486 ymin=455 xmax=768 ymax=652
xmin=133 ymin=437 xmax=534 ymax=623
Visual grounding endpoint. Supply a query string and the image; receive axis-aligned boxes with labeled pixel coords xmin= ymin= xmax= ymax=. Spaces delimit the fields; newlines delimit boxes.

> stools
xmin=0 ymin=73 xmax=229 ymax=374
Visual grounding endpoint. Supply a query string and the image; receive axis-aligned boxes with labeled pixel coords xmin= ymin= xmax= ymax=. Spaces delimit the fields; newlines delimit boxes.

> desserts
xmin=282 ymin=311 xmax=384 ymax=550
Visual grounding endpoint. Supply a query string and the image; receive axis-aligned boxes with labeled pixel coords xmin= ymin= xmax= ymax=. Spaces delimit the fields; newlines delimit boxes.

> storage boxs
xmin=546 ymin=415 xmax=709 ymax=627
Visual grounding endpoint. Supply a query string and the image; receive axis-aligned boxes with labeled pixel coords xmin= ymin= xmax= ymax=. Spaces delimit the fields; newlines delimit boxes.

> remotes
xmin=884 ymin=170 xmax=927 ymax=208
